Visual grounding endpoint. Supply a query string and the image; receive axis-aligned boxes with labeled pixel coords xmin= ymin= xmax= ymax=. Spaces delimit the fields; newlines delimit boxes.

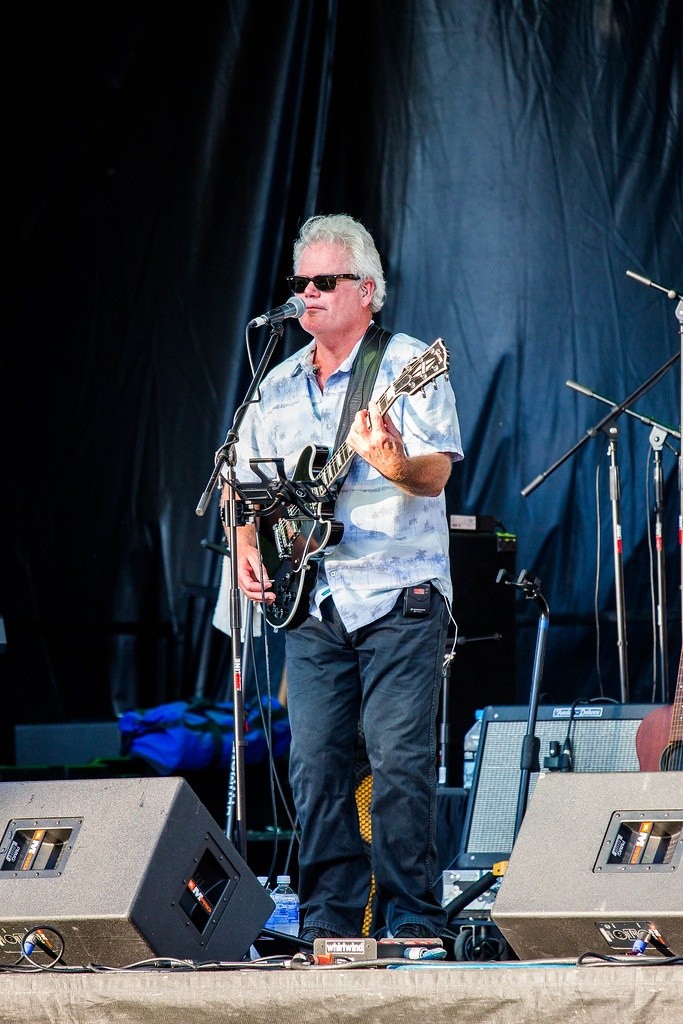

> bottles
xmin=269 ymin=876 xmax=301 ymax=962
xmin=249 ymin=876 xmax=273 ymax=961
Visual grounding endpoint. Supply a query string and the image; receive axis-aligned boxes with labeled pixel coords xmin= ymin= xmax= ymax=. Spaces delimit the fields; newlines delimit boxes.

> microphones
xmin=248 ymin=296 xmax=306 ymax=329
xmin=558 ymin=738 xmax=572 ymax=773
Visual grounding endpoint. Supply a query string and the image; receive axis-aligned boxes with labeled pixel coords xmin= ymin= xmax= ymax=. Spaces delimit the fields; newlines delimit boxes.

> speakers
xmin=491 ymin=770 xmax=683 ymax=961
xmin=0 ymin=776 xmax=276 ymax=965
xmin=459 ymin=703 xmax=675 ymax=868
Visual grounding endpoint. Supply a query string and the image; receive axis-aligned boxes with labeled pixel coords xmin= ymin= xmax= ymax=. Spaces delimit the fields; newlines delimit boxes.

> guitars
xmin=634 ymin=639 xmax=683 ymax=771
xmin=237 ymin=334 xmax=450 ymax=629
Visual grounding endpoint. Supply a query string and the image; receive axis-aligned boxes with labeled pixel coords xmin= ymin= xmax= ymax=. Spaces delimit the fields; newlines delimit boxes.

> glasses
xmin=285 ymin=273 xmax=361 ymax=294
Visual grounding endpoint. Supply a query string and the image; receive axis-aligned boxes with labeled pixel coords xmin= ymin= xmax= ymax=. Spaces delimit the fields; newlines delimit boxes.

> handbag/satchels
xmin=119 ymin=693 xmax=290 ymax=772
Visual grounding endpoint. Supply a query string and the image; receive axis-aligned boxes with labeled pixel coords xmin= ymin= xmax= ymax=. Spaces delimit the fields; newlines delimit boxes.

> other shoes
xmin=299 ymin=927 xmax=331 ymax=954
xmin=393 ymin=924 xmax=437 ymax=939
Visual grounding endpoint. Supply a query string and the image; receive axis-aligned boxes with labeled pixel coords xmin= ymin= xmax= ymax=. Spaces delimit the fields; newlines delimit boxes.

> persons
xmin=219 ymin=210 xmax=468 ymax=961
